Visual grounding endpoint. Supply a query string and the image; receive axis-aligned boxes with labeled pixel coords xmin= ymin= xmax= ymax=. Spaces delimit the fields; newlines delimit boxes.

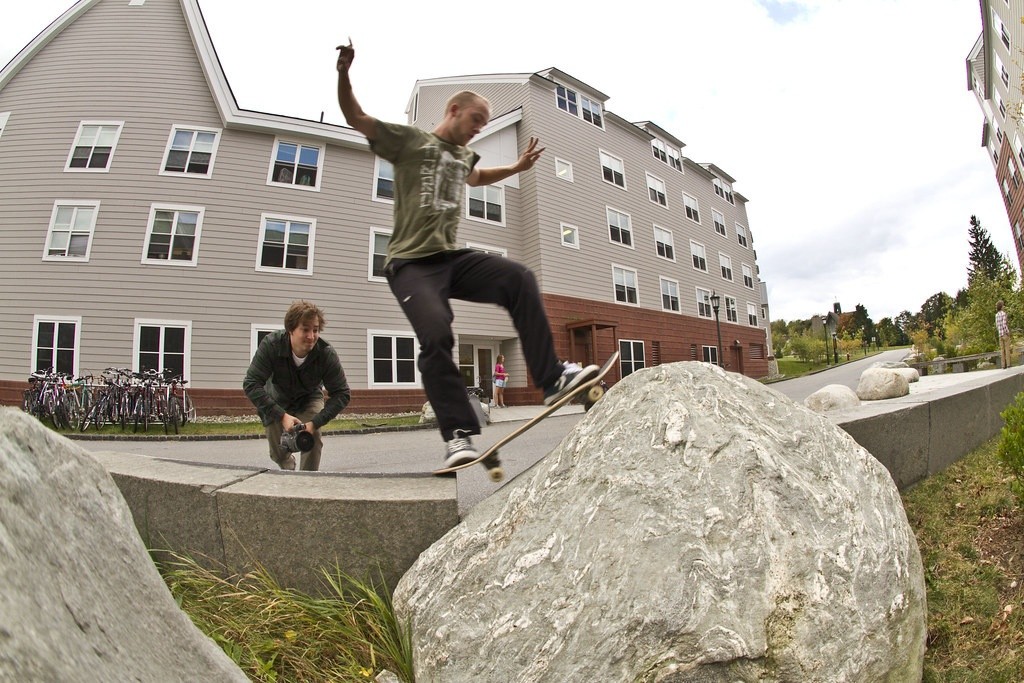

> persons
xmin=995 ymin=300 xmax=1013 ymax=369
xmin=336 ymin=37 xmax=601 ymax=471
xmin=243 ymin=299 xmax=350 ymax=471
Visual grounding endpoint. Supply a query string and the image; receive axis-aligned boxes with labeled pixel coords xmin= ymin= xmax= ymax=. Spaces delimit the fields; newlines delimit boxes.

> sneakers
xmin=444 ymin=429 xmax=480 ymax=467
xmin=543 ymin=360 xmax=600 ymax=405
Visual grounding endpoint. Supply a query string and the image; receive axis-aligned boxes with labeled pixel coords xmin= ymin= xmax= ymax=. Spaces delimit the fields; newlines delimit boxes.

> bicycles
xmin=23 ymin=366 xmax=197 ymax=436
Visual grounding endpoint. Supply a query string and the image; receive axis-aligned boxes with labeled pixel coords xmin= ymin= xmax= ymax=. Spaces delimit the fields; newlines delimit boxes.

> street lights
xmin=821 ymin=316 xmax=830 ymax=365
xmin=709 ymin=290 xmax=725 ymax=368
xmin=861 ymin=324 xmax=867 ymax=356
xmin=875 ymin=328 xmax=879 ymax=352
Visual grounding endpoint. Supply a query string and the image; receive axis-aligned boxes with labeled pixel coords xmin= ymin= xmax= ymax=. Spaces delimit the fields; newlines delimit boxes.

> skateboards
xmin=435 ymin=348 xmax=621 ymax=482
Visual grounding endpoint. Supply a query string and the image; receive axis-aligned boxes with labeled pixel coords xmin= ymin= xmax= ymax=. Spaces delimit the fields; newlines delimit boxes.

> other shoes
xmin=495 ymin=405 xmax=501 ymax=409
xmin=501 ymin=405 xmax=507 ymax=408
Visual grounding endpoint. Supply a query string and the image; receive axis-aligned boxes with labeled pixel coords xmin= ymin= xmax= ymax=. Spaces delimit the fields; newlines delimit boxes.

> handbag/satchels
xmin=492 ymin=375 xmax=496 ymax=383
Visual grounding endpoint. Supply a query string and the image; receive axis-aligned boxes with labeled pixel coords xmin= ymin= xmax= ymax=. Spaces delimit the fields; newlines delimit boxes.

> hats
xmin=505 ymin=377 xmax=508 ymax=384
